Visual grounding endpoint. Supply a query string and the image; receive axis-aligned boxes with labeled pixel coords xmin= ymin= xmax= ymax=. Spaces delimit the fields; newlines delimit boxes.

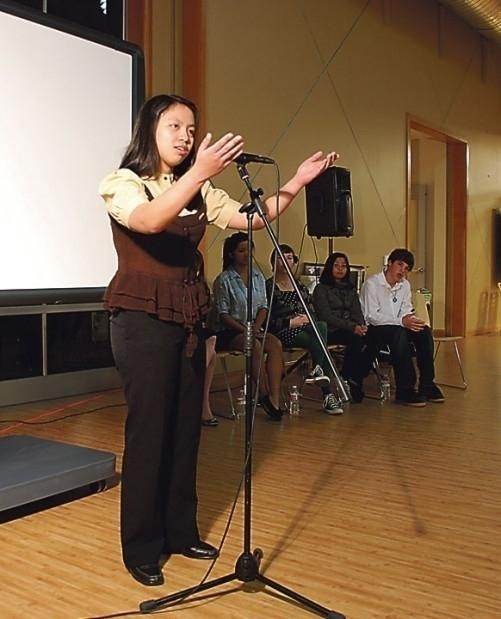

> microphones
xmin=230 ymin=144 xmax=265 ymax=166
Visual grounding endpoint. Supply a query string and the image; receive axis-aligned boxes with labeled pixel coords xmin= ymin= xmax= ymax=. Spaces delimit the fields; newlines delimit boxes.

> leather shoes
xmin=260 ymin=395 xmax=283 ymax=421
xmin=128 ymin=564 xmax=164 ymax=586
xmin=182 ymin=541 xmax=218 ymax=559
xmin=201 ymin=417 xmax=219 ymax=426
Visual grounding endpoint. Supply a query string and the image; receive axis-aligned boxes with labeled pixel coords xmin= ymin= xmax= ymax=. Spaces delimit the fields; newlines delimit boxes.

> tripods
xmin=137 ymin=210 xmax=352 ymax=618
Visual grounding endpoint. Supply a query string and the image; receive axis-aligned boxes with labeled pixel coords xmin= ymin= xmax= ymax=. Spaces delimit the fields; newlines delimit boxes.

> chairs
xmin=203 ymin=293 xmax=468 ymax=423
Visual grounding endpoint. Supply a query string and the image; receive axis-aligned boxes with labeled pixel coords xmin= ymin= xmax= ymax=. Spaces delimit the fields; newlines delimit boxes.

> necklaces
xmin=389 ymin=289 xmax=397 ymax=302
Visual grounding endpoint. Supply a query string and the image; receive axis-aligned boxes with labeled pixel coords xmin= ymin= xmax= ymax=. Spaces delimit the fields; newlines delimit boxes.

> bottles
xmin=381 ymin=375 xmax=391 ymax=400
xmin=235 ymin=390 xmax=246 ymax=416
xmin=343 ymin=380 xmax=351 ymax=402
xmin=290 ymin=385 xmax=300 ymax=415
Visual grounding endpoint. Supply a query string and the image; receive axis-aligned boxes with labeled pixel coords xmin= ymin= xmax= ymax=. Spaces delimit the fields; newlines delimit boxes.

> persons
xmin=205 ymin=326 xmax=221 ymax=426
xmin=214 ymin=232 xmax=283 ymax=418
xmin=313 ymin=253 xmax=382 ymax=403
xmin=101 ymin=92 xmax=342 ymax=586
xmin=358 ymin=248 xmax=445 ymax=406
xmin=265 ymin=244 xmax=344 ymax=415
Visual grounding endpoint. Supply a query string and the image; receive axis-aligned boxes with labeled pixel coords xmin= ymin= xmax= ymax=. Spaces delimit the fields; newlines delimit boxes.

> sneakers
xmin=418 ymin=383 xmax=445 ymax=402
xmin=303 ymin=364 xmax=330 ymax=386
xmin=395 ymin=388 xmax=426 ymax=407
xmin=342 ymin=376 xmax=364 ymax=403
xmin=323 ymin=393 xmax=344 ymax=414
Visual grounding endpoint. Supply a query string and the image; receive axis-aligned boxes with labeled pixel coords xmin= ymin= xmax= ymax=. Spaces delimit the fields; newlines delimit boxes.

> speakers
xmin=305 ymin=165 xmax=353 ymax=238
